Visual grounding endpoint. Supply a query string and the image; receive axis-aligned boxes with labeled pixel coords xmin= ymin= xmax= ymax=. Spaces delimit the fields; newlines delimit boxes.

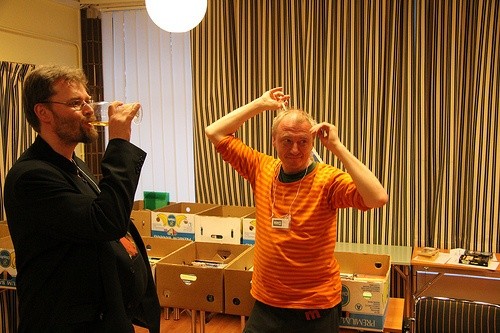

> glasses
xmin=37 ymin=96 xmax=94 ymax=110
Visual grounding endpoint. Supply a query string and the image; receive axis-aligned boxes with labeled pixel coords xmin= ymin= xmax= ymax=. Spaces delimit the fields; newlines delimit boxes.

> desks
xmin=412 ymin=245 xmax=500 ymax=306
xmin=335 ymin=242 xmax=411 ymax=318
xmin=382 ymin=297 xmax=405 ymax=333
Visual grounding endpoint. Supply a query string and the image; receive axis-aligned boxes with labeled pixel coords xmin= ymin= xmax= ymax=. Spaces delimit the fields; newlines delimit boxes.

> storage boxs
xmin=0 ymin=199 xmax=391 ymax=333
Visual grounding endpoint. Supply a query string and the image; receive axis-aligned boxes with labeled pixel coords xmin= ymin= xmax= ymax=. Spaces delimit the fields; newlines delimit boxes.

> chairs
xmin=410 ymin=297 xmax=500 ymax=333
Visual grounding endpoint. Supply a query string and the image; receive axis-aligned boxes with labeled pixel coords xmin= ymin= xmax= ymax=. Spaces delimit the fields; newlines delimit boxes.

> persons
xmin=205 ymin=87 xmax=389 ymax=333
xmin=4 ymin=64 xmax=162 ymax=333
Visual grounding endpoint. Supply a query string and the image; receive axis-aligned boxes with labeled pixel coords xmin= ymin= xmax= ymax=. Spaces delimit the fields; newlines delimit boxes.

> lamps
xmin=144 ymin=0 xmax=207 ymax=34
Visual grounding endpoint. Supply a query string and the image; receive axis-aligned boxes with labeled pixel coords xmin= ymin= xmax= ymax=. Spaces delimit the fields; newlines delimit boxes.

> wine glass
xmin=86 ymin=102 xmax=144 ymax=126
